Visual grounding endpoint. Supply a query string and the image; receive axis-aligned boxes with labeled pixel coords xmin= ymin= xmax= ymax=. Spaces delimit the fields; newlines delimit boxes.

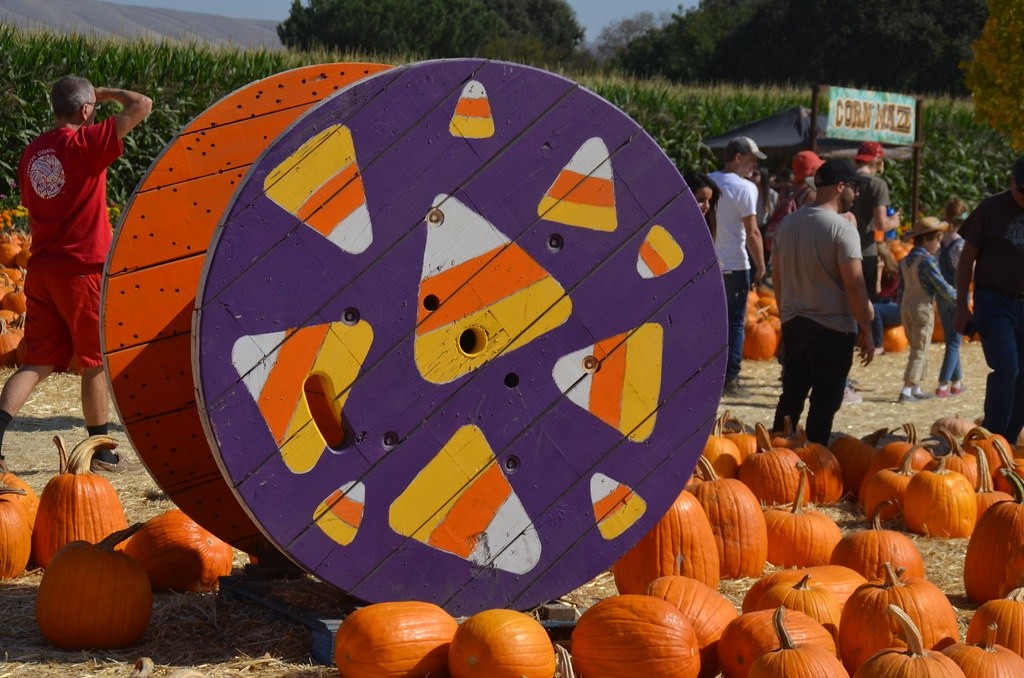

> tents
xmin=667 ymin=106 xmax=915 ymax=224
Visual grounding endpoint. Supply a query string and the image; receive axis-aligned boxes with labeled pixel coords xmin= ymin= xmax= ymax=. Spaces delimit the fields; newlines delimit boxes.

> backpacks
xmin=764 ymin=183 xmax=810 ymax=252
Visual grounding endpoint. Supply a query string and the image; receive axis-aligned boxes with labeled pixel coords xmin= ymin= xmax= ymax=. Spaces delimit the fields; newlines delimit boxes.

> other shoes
xmin=951 ymin=382 xmax=970 ymax=394
xmin=935 ymin=387 xmax=951 ymax=397
xmin=722 ymin=377 xmax=750 ymax=400
xmin=898 ymin=392 xmax=920 ymax=403
xmin=90 ymin=453 xmax=144 ymax=476
xmin=840 ymin=388 xmax=863 ymax=403
xmin=911 ymin=392 xmax=934 ymax=400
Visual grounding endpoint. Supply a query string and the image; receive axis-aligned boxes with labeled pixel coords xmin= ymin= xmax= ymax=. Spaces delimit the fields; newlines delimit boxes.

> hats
xmin=724 ymin=136 xmax=768 ymax=160
xmin=792 ymin=151 xmax=826 ymax=184
xmin=814 ymin=158 xmax=872 ymax=188
xmin=853 ymin=142 xmax=885 ymax=162
xmin=904 ymin=217 xmax=949 ymax=239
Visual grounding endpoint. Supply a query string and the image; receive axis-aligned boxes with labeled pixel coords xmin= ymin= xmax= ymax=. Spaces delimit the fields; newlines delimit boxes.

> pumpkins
xmin=0 ymin=209 xmax=33 ymax=363
xmin=336 ymin=410 xmax=1024 ymax=678
xmin=882 ymin=283 xmax=981 ymax=351
xmin=0 ymin=434 xmax=234 ymax=647
xmin=887 ymin=239 xmax=914 ymax=260
xmin=745 ymin=283 xmax=781 ymax=360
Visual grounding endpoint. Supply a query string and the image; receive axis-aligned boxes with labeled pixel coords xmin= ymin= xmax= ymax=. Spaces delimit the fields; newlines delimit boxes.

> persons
xmin=933 ymin=196 xmax=976 ymax=398
xmin=746 ymin=142 xmax=901 ymax=355
xmin=898 ymin=217 xmax=958 ymax=404
xmin=0 ymin=75 xmax=152 ymax=474
xmin=770 ymin=158 xmax=875 ymax=447
xmin=685 ymin=173 xmax=723 ymax=246
xmin=707 ymin=137 xmax=766 ymax=398
xmin=956 ymin=158 xmax=1024 ymax=445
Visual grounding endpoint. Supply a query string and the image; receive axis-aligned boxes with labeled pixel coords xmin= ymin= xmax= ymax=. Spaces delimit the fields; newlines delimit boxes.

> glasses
xmin=842 ymin=182 xmax=859 ymax=193
xmin=80 ymin=101 xmax=103 ymax=110
